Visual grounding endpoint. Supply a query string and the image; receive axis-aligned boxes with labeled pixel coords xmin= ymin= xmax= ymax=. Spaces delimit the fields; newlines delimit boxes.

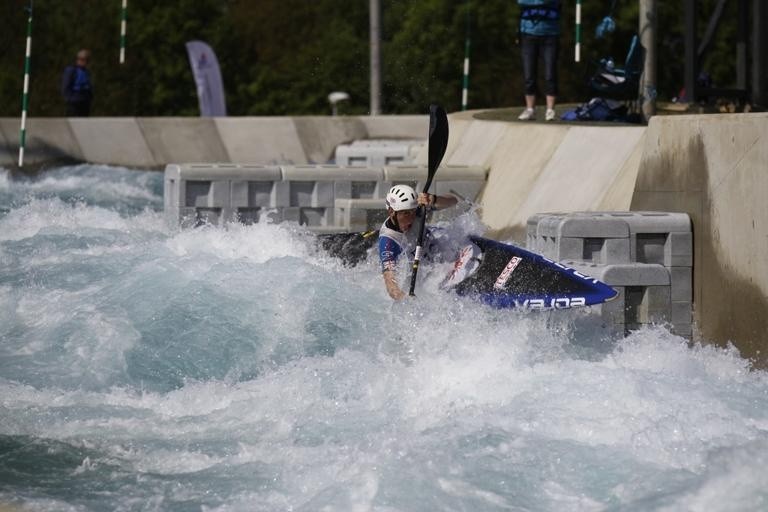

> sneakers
xmin=517 ymin=109 xmax=537 ymax=120
xmin=544 ymin=110 xmax=557 ymax=122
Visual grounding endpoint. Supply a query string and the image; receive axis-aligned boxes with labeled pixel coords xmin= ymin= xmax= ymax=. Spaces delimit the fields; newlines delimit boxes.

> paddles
xmin=407 ymin=103 xmax=448 ymax=298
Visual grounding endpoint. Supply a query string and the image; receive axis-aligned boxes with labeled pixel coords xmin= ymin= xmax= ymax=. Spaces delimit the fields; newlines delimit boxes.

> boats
xmin=309 ymin=228 xmax=626 ymax=318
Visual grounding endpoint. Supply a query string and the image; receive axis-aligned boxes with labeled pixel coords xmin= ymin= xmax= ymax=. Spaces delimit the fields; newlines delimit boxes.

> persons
xmin=515 ymin=0 xmax=562 ymax=120
xmin=377 ymin=187 xmax=582 ymax=300
xmin=62 ymin=48 xmax=93 ymax=116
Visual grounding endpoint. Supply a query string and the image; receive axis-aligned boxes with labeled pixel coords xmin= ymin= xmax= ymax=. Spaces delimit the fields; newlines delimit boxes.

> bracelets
xmin=432 ymin=193 xmax=437 ymax=212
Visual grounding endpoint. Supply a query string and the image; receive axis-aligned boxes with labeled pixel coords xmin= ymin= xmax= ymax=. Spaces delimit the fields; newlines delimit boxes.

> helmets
xmin=385 ymin=184 xmax=421 ymax=213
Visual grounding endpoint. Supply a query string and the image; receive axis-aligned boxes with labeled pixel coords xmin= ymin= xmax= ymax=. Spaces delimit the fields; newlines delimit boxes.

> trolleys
xmin=572 ymin=13 xmax=644 ymax=126
